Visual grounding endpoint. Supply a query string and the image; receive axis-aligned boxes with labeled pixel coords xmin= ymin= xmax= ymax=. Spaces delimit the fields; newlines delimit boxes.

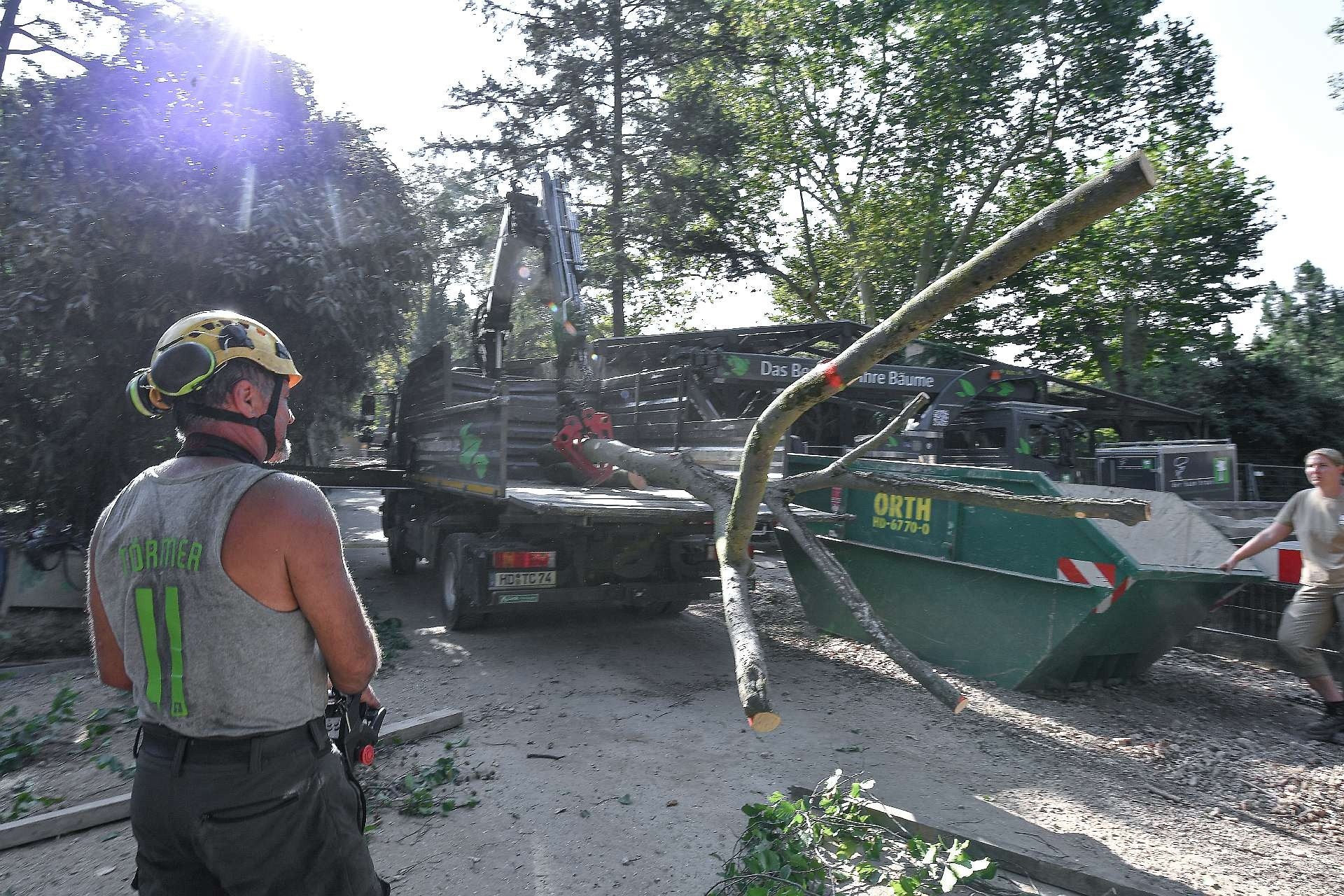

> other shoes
xmin=1332 ymin=731 xmax=1344 ymax=744
xmin=1304 ymin=699 xmax=1344 ymax=738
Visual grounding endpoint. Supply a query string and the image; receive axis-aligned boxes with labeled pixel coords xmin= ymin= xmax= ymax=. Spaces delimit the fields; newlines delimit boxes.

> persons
xmin=87 ymin=309 xmax=392 ymax=896
xmin=1218 ymin=449 xmax=1344 ymax=735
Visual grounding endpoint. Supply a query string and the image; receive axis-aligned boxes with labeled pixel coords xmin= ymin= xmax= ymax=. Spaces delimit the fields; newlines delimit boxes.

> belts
xmin=144 ymin=713 xmax=328 ymax=762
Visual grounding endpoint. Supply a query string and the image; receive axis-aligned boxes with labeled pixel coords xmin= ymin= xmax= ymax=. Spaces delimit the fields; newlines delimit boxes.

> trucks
xmin=350 ymin=338 xmax=788 ymax=633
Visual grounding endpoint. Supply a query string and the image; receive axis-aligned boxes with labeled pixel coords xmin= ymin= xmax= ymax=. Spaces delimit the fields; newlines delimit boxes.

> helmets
xmin=150 ymin=310 xmax=303 ymax=397
